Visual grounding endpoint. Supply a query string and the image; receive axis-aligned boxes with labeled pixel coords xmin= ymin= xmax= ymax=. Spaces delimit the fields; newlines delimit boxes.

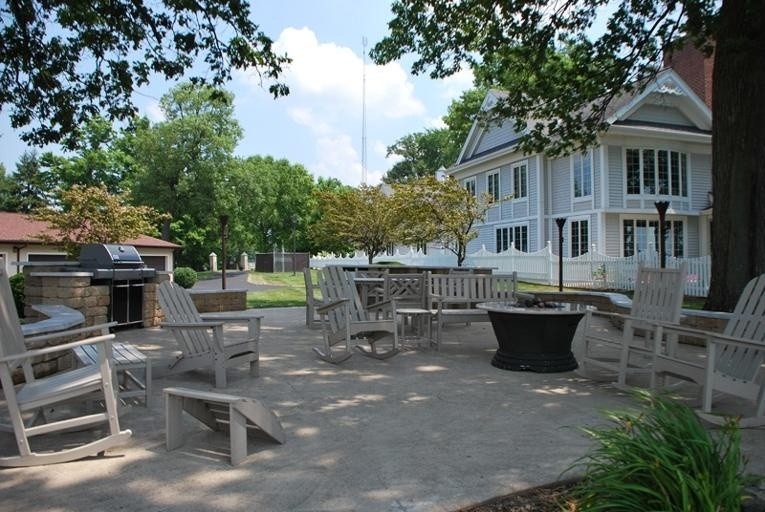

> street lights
xmin=653 ymin=201 xmax=671 ymax=268
xmin=291 ymin=214 xmax=297 ymax=277
xmin=555 ymin=217 xmax=567 ymax=291
xmin=219 ymin=215 xmax=229 ymax=289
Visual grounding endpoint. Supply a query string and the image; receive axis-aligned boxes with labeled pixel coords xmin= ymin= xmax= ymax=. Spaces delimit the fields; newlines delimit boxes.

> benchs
xmin=429 ymin=271 xmax=519 ymax=350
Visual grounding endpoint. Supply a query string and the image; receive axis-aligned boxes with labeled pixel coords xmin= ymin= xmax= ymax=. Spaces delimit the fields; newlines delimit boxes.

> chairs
xmin=574 ymin=265 xmax=692 ymax=394
xmin=0 ymin=254 xmax=133 ymax=468
xmin=303 ymin=266 xmax=429 ymax=364
xmin=649 ymin=273 xmax=765 ymax=428
xmin=157 ymin=280 xmax=266 ymax=390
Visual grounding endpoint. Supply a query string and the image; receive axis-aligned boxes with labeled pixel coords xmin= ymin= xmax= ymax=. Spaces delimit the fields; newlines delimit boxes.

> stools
xmin=70 ymin=337 xmax=153 ymax=408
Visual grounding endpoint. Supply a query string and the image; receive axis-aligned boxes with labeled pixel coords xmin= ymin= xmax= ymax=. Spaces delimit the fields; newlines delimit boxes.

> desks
xmin=477 ymin=302 xmax=597 ymax=373
